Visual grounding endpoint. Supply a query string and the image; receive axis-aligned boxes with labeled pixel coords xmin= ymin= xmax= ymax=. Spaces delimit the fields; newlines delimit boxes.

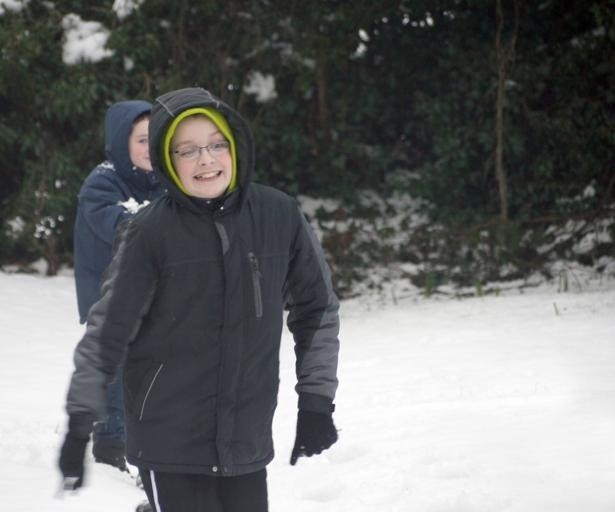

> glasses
xmin=176 ymin=139 xmax=230 ymax=162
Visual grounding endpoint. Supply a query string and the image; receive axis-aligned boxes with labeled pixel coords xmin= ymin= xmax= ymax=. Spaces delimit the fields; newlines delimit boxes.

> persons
xmin=73 ymin=102 xmax=169 ymax=479
xmin=59 ymin=87 xmax=339 ymax=512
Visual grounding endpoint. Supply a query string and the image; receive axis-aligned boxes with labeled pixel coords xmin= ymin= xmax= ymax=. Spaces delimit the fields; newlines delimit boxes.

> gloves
xmin=288 ymin=407 xmax=338 ymax=466
xmin=58 ymin=411 xmax=92 ymax=491
